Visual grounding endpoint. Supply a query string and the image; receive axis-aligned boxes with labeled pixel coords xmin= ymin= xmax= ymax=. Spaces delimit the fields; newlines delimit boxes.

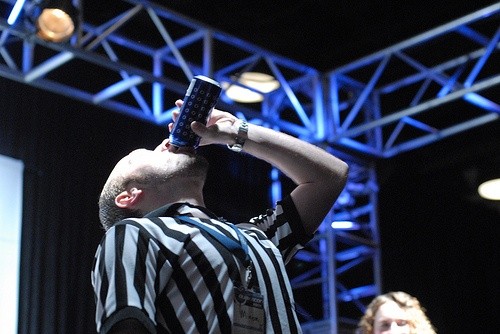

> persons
xmin=353 ymin=292 xmax=437 ymax=334
xmin=91 ymin=99 xmax=350 ymax=334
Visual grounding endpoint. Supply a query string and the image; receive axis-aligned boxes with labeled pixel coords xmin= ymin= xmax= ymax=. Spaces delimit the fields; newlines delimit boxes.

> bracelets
xmin=227 ymin=119 xmax=248 ymax=153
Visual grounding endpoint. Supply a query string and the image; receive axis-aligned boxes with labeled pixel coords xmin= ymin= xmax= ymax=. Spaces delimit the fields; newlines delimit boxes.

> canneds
xmin=168 ymin=75 xmax=222 ymax=151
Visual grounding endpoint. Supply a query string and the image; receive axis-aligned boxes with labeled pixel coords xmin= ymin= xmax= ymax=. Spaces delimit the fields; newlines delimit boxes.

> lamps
xmin=35 ymin=1 xmax=78 ymax=45
